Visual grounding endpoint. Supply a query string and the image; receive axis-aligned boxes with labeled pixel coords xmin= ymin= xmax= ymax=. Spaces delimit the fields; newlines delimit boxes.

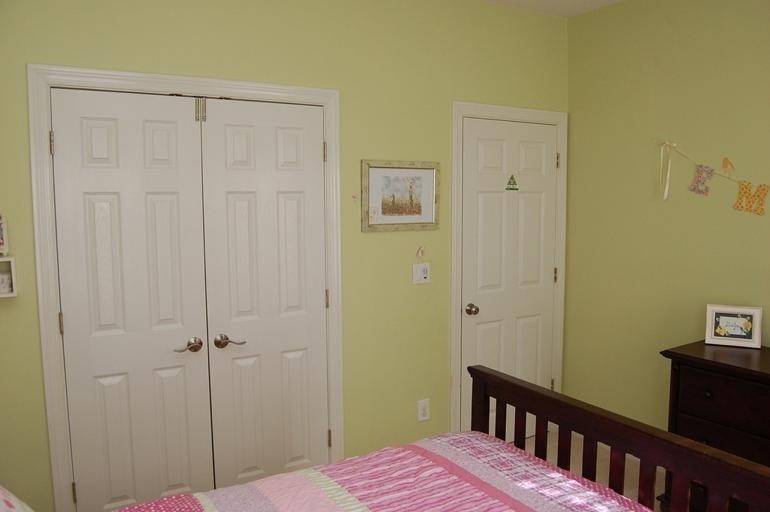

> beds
xmin=0 ymin=364 xmax=768 ymax=512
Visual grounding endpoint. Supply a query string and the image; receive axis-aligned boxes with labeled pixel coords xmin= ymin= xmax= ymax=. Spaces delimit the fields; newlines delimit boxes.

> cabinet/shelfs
xmin=656 ymin=338 xmax=770 ymax=511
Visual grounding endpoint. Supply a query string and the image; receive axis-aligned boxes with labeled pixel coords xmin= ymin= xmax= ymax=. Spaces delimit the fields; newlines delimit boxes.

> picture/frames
xmin=0 ymin=214 xmax=18 ymax=298
xmin=705 ymin=302 xmax=763 ymax=350
xmin=360 ymin=159 xmax=440 ymax=234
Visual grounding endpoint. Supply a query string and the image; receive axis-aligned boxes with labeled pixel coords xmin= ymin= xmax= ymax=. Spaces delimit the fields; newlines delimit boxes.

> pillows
xmin=0 ymin=487 xmax=33 ymax=512
